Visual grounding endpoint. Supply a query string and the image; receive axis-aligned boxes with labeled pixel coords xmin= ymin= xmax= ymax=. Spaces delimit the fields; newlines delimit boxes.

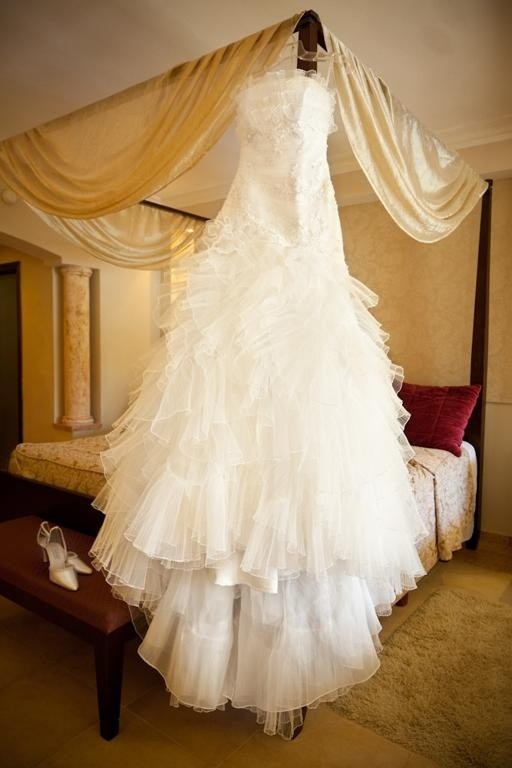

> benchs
xmin=0 ymin=515 xmax=153 ymax=739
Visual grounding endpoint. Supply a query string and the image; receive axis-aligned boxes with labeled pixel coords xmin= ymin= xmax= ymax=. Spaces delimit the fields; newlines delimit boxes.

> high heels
xmin=36 ymin=521 xmax=78 ymax=593
xmin=64 ymin=549 xmax=93 ymax=575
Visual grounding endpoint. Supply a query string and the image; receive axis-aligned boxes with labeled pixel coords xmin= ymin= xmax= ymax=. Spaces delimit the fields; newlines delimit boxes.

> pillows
xmin=389 ymin=379 xmax=481 ymax=457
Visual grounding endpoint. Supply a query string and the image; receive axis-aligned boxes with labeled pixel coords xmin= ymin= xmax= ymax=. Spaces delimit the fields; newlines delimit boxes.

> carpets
xmin=327 ymin=585 xmax=512 ymax=768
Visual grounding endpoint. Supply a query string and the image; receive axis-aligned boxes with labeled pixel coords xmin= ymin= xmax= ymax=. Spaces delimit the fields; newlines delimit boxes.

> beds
xmin=0 ymin=12 xmax=496 ymax=738
